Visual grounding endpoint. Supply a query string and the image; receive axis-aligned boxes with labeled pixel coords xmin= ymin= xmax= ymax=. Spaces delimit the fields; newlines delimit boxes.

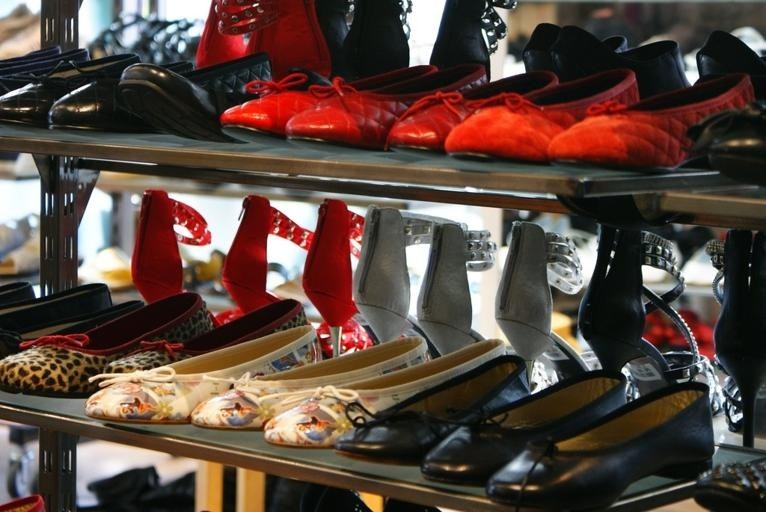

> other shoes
xmin=264 ymin=338 xmax=506 ymax=447
xmin=0 ymin=283 xmax=307 ymax=396
xmin=84 ymin=325 xmax=322 ymax=424
xmin=191 ymin=335 xmax=432 ymax=429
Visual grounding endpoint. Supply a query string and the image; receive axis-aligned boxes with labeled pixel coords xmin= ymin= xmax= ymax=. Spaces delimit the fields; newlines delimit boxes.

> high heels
xmin=705 ymin=228 xmax=766 ymax=450
xmin=417 ymin=222 xmax=496 ymax=356
xmin=130 ymin=188 xmax=212 ymax=304
xmin=496 ymin=220 xmax=603 ymax=387
xmin=301 ymin=198 xmax=374 ymax=359
xmin=352 ymin=205 xmax=467 ymax=345
xmin=578 ymin=223 xmax=722 ymax=417
xmin=220 ymin=196 xmax=313 ymax=311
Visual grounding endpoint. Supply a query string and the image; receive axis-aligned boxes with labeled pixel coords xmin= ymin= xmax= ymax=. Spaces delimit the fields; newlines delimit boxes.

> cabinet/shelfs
xmin=0 ymin=117 xmax=766 ymax=512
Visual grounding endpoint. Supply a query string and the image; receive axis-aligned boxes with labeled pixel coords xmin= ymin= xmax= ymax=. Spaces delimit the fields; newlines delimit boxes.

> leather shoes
xmin=695 ymin=456 xmax=765 ymax=511
xmin=333 ymin=355 xmax=531 ymax=465
xmin=1 ymin=0 xmax=766 ymax=183
xmin=422 ymin=369 xmax=628 ymax=486
xmin=486 ymin=382 xmax=713 ymax=512
